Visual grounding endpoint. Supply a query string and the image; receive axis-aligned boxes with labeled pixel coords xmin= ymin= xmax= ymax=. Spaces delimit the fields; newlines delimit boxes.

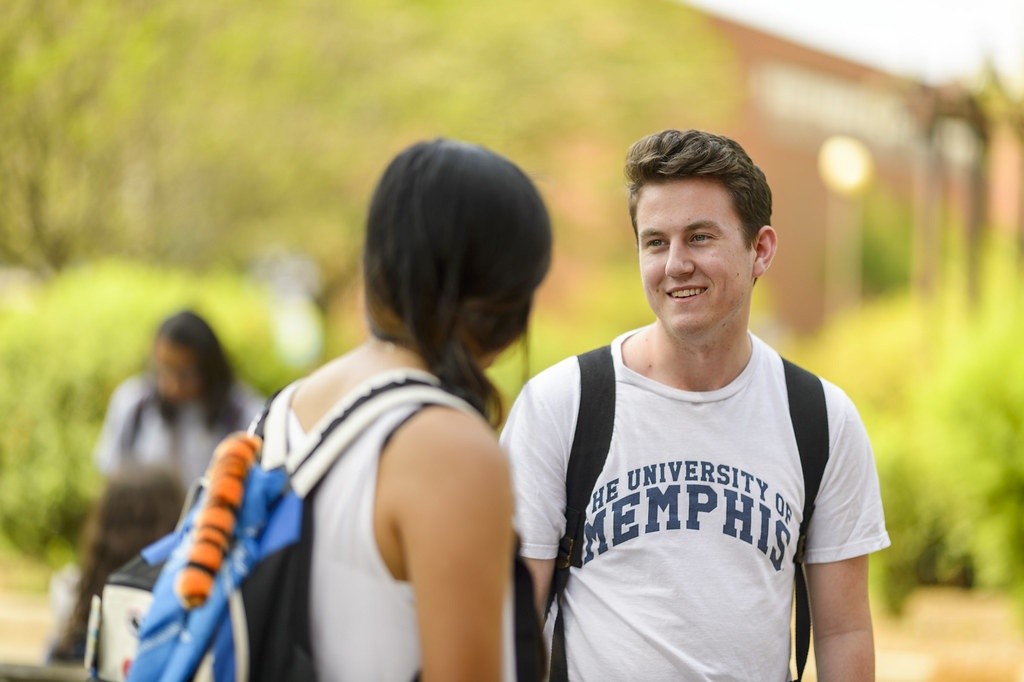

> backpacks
xmin=85 ymin=366 xmax=476 ymax=682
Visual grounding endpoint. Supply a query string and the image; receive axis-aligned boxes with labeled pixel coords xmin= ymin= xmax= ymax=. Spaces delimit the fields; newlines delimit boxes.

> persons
xmin=500 ymin=130 xmax=893 ymax=682
xmin=49 ymin=311 xmax=261 ymax=669
xmin=246 ymin=137 xmax=552 ymax=682
